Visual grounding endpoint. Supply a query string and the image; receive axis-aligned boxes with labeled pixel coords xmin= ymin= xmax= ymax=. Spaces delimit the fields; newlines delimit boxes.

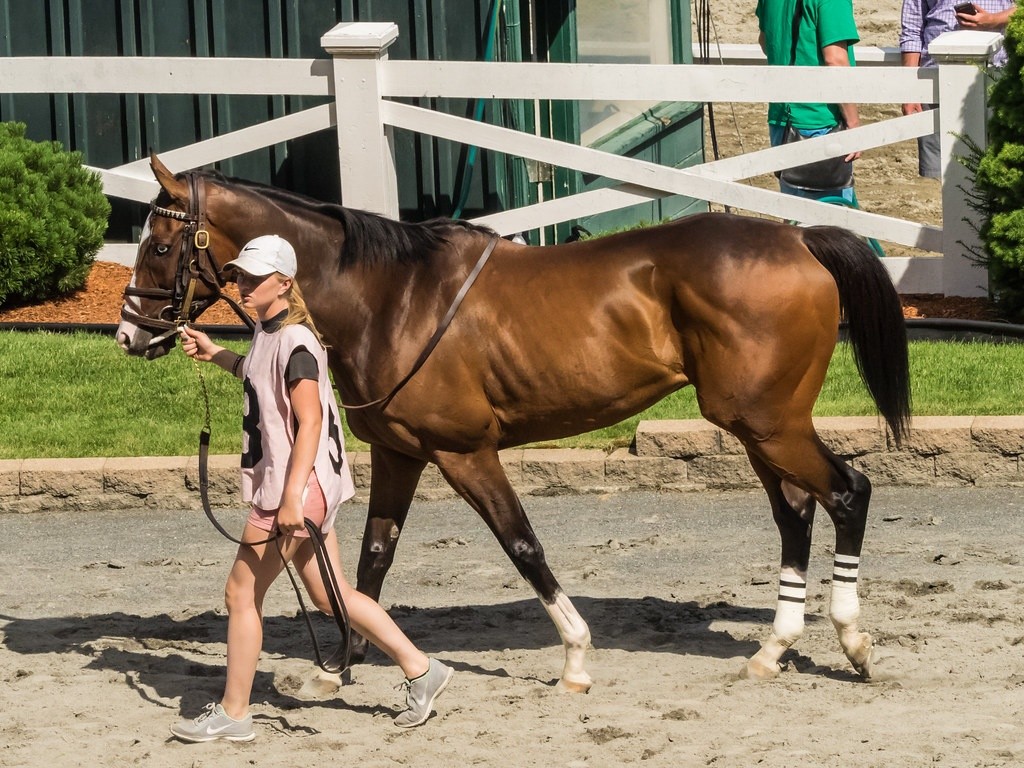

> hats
xmin=222 ymin=233 xmax=297 ymax=280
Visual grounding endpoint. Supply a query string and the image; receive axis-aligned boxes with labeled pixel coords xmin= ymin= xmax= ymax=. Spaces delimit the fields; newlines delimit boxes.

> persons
xmin=755 ymin=0 xmax=863 ymax=223
xmin=169 ymin=233 xmax=455 ymax=745
xmin=900 ymin=0 xmax=1019 ymax=180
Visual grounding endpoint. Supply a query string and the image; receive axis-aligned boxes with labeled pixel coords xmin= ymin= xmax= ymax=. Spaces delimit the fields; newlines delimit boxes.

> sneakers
xmin=395 ymin=657 xmax=454 ymax=729
xmin=169 ymin=700 xmax=256 ymax=744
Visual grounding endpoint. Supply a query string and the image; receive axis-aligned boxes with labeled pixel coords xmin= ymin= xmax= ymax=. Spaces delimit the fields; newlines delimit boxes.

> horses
xmin=113 ymin=144 xmax=917 ymax=704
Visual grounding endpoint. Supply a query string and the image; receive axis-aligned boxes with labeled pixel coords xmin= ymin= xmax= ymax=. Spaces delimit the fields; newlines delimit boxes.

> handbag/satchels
xmin=773 ymin=116 xmax=858 ymax=194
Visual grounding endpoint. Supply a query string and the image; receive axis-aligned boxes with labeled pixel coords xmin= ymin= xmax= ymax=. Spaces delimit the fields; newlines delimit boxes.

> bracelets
xmin=232 ymin=354 xmax=245 ymax=378
